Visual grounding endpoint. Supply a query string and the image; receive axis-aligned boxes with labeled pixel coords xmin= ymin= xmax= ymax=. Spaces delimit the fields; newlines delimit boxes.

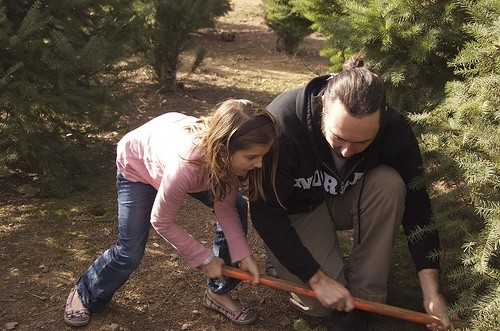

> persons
xmin=250 ymin=48 xmax=466 ymax=330
xmin=63 ymin=97 xmax=288 ymax=327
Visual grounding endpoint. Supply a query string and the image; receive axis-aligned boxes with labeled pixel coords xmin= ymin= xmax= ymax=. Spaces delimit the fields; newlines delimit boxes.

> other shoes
xmin=203 ymin=287 xmax=257 ymax=325
xmin=62 ymin=286 xmax=90 ymax=326
